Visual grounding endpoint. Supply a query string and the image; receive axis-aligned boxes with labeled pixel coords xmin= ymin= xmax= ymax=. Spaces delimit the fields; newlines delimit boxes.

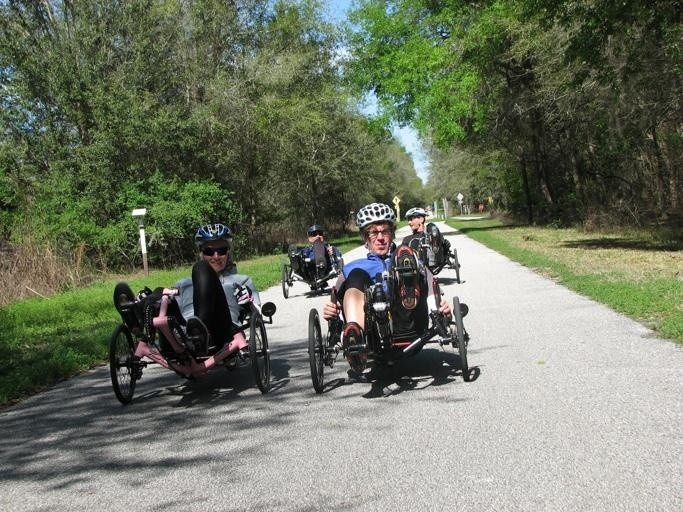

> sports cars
xmin=305 ymin=263 xmax=469 ymax=393
xmin=396 ymin=232 xmax=461 ymax=284
xmin=279 ymin=243 xmax=343 ymax=299
xmin=106 ymin=283 xmax=277 ymax=404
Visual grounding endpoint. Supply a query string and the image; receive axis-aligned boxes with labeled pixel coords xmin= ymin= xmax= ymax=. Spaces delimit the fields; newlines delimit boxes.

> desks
xmin=131 ymin=207 xmax=150 ymax=278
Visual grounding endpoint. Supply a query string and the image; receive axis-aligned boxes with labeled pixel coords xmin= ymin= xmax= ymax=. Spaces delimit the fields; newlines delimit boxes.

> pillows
xmin=356 ymin=202 xmax=397 ymax=230
xmin=307 ymin=225 xmax=324 ymax=233
xmin=405 ymin=207 xmax=428 ymax=219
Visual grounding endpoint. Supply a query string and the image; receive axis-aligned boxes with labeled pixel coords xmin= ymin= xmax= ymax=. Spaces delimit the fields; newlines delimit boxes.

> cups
xmin=309 ymin=231 xmax=324 ymax=237
xmin=364 ymin=228 xmax=392 ymax=239
xmin=199 ymin=244 xmax=230 ymax=256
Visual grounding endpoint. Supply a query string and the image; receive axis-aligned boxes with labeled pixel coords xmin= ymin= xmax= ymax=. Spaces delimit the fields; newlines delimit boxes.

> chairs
xmin=314 ymin=241 xmax=327 ymax=272
xmin=113 ymin=284 xmax=144 ymax=337
xmin=186 ymin=317 xmax=210 ymax=364
xmin=287 ymin=244 xmax=300 ymax=271
xmin=431 ymin=224 xmax=440 ymax=254
xmin=342 ymin=322 xmax=367 ymax=375
xmin=195 ymin=223 xmax=233 ymax=243
xmin=394 ymin=245 xmax=423 ymax=310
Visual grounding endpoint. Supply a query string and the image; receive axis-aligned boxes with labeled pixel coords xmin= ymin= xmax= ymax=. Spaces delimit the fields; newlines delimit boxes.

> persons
xmin=322 ymin=200 xmax=452 ymax=374
xmin=286 ymin=223 xmax=342 ymax=290
xmin=399 ymin=206 xmax=450 ymax=275
xmin=133 ymin=222 xmax=263 ymax=366
